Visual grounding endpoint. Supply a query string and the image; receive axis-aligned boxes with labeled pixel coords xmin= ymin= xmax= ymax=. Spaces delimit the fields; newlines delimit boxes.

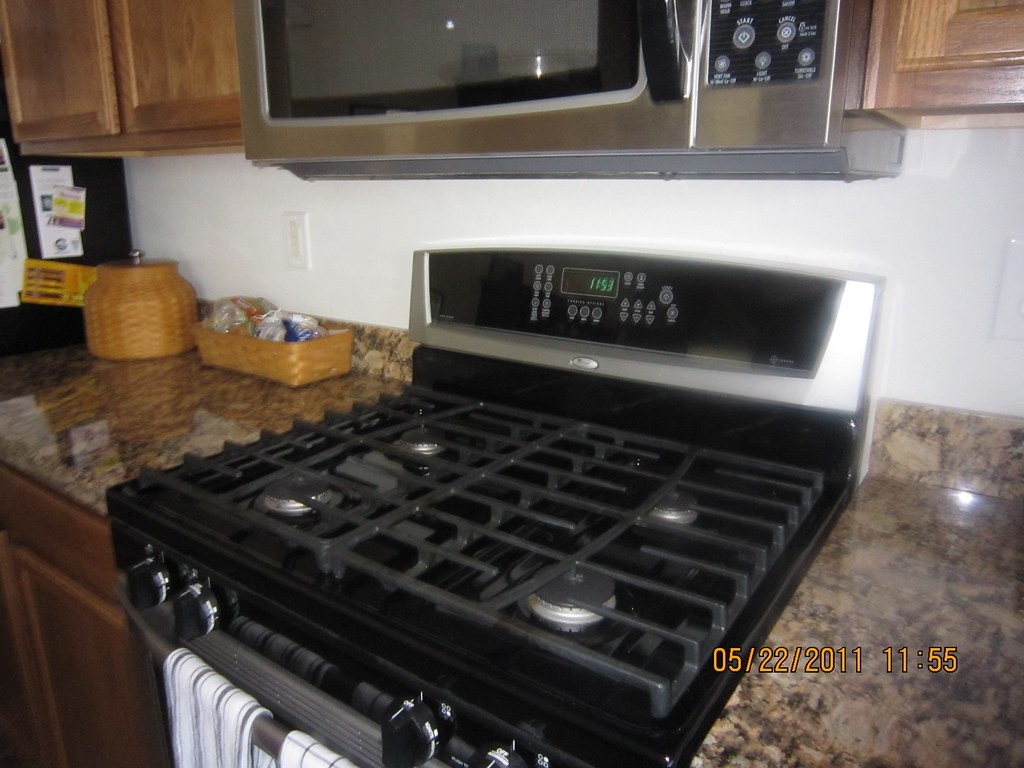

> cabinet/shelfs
xmin=0 ymin=300 xmax=412 ymax=768
xmin=863 ymin=0 xmax=1024 ymax=130
xmin=0 ymin=0 xmax=248 ymax=156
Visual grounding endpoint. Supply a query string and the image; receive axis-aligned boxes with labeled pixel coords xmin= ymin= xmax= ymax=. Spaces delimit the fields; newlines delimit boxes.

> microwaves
xmin=227 ymin=0 xmax=907 ymax=181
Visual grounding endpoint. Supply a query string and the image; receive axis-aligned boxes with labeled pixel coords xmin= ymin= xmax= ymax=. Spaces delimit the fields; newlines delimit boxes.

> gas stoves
xmin=103 ymin=241 xmax=887 ymax=768
